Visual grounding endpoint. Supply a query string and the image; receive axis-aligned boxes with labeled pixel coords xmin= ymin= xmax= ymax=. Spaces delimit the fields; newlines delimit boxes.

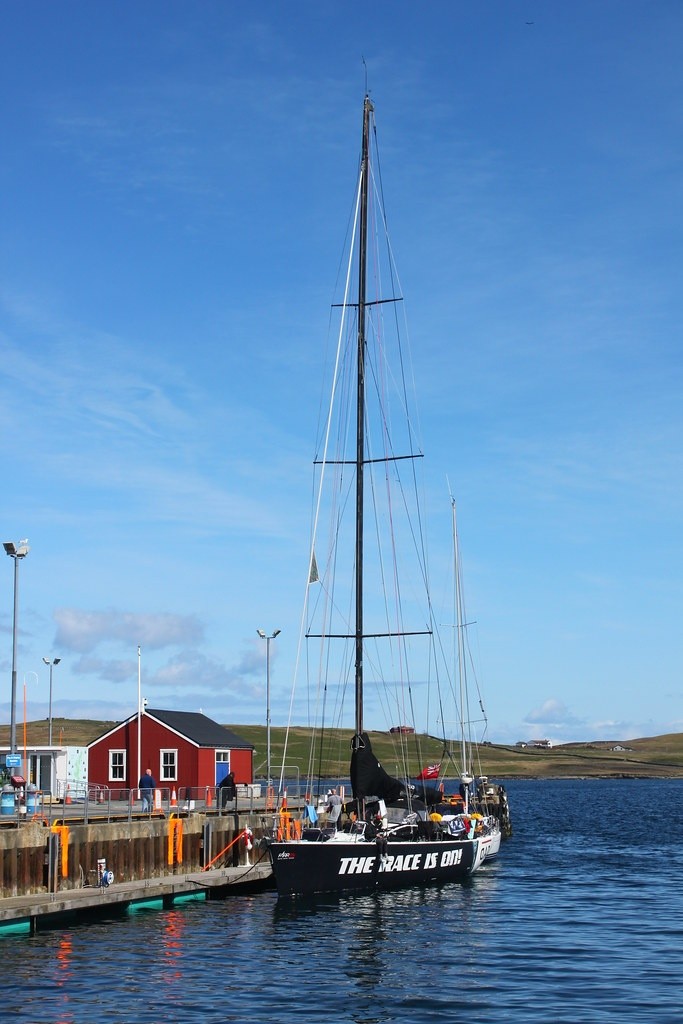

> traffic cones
xmin=97 ymin=788 xmax=107 ymax=805
xmin=126 ymin=785 xmax=137 ymax=806
xmin=205 ymin=789 xmax=213 ymax=807
xmin=65 ymin=784 xmax=72 ymax=804
xmin=170 ymin=786 xmax=178 ymax=807
xmin=282 ymin=788 xmax=287 ymax=808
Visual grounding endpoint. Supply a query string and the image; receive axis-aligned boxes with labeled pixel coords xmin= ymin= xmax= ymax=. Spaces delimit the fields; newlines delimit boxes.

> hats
xmin=375 ymin=815 xmax=382 ymax=819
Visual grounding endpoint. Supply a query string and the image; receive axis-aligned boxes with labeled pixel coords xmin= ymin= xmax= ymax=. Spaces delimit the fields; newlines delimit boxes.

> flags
xmin=418 ymin=763 xmax=441 ymax=780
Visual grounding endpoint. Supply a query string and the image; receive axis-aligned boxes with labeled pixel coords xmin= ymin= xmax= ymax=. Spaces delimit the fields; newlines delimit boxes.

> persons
xmin=364 ymin=819 xmax=392 ymax=863
xmin=216 ymin=772 xmax=236 ymax=810
xmin=325 ymin=788 xmax=343 ymax=832
xmin=139 ymin=769 xmax=156 ymax=813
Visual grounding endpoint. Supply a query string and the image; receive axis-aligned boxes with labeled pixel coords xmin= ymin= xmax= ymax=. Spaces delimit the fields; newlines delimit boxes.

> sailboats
xmin=261 ymin=91 xmax=493 ymax=895
xmin=433 ymin=494 xmax=512 ymax=864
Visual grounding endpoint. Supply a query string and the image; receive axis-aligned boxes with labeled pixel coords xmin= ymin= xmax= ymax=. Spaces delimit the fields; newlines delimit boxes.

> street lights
xmin=43 ymin=657 xmax=61 ymax=746
xmin=3 ymin=542 xmax=29 ymax=777
xmin=256 ymin=629 xmax=281 ymax=785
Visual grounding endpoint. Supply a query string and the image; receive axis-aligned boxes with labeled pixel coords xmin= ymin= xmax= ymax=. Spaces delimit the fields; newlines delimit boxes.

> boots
xmin=379 ymin=853 xmax=392 ymax=863
